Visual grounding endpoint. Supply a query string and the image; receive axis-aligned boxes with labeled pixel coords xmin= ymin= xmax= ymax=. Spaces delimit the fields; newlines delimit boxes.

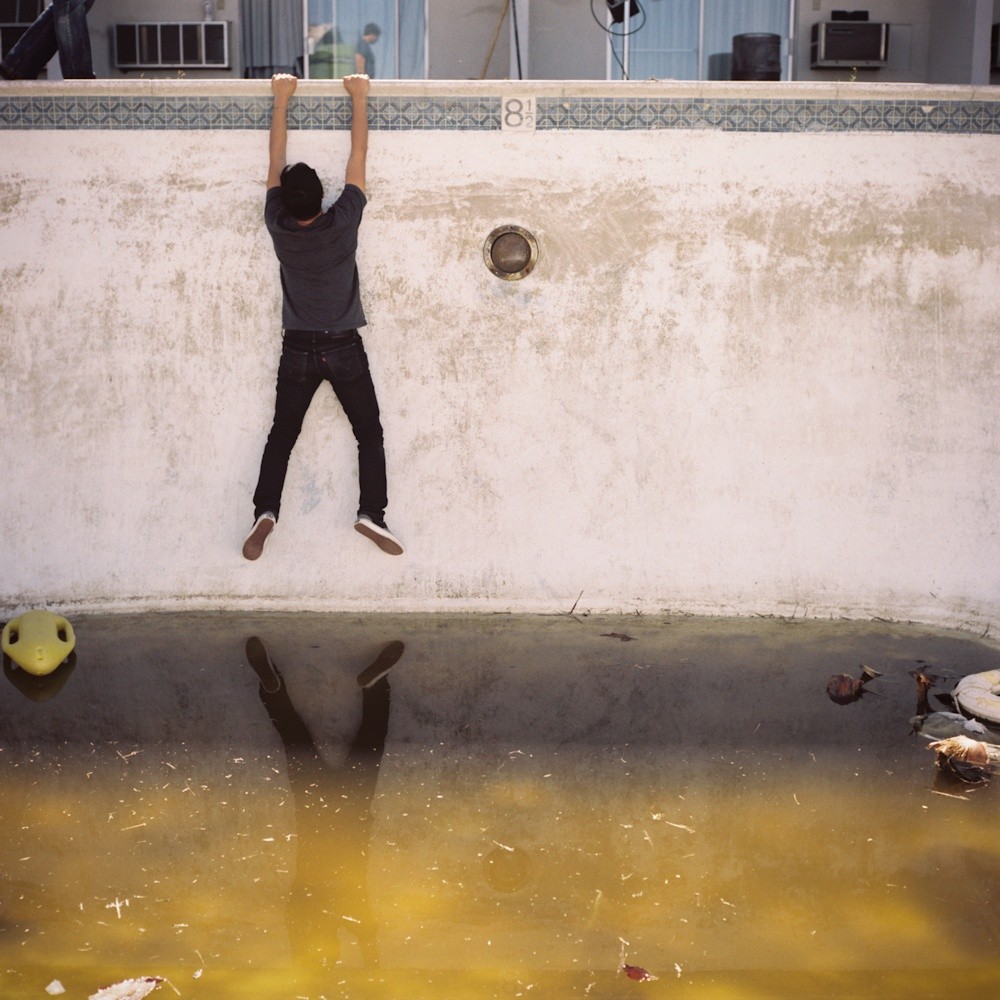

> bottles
xmin=203 ymin=0 xmax=214 ymax=21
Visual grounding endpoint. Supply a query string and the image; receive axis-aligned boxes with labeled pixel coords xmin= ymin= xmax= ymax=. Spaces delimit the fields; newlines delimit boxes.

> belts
xmin=285 ymin=330 xmax=357 ymax=338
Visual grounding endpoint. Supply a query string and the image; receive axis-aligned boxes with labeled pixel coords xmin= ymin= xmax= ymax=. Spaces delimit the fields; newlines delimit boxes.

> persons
xmin=355 ymin=23 xmax=382 ymax=79
xmin=244 ymin=633 xmax=407 ymax=970
xmin=240 ymin=73 xmax=405 ymax=561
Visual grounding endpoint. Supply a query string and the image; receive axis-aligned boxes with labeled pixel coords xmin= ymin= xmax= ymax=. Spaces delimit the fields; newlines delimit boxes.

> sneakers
xmin=241 ymin=511 xmax=275 ymax=560
xmin=357 ymin=640 xmax=405 ymax=688
xmin=246 ymin=636 xmax=280 ymax=693
xmin=354 ymin=512 xmax=404 ymax=556
